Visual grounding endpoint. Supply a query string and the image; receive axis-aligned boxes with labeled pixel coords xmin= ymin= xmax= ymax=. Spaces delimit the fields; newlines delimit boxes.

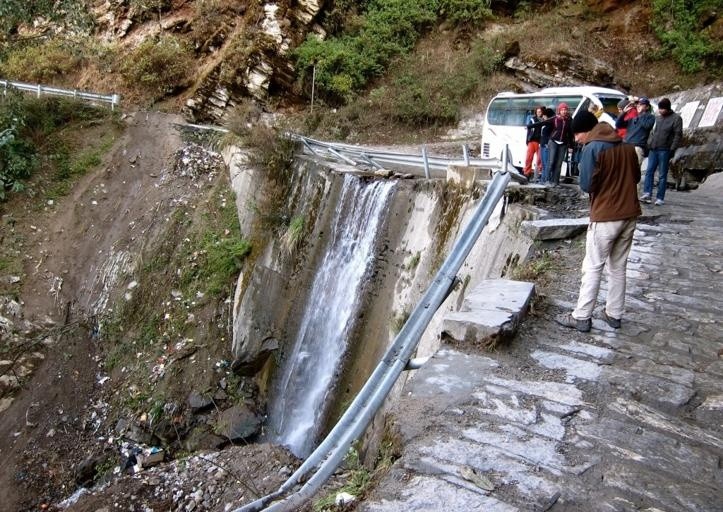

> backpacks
xmin=605 ymin=112 xmax=617 ymax=121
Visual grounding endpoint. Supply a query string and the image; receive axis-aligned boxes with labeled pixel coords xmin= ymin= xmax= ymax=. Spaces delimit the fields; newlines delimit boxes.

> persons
xmin=554 ymin=112 xmax=642 ymax=332
xmin=523 ymin=96 xmax=682 ymax=206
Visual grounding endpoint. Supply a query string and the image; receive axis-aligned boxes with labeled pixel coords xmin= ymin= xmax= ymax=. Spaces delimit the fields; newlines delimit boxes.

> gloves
xmin=668 ymin=150 xmax=674 ymax=157
xmin=644 ymin=150 xmax=649 ymax=157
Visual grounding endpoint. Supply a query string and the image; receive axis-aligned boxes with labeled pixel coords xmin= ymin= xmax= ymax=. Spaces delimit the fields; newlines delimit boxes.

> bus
xmin=480 ymin=86 xmax=628 ymax=178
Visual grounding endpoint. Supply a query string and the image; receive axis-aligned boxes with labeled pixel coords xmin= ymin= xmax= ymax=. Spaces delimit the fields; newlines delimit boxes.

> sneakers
xmin=592 ymin=305 xmax=621 ymax=328
xmin=557 ymin=313 xmax=592 ymax=332
xmin=655 ymin=198 xmax=665 ymax=206
xmin=640 ymin=193 xmax=652 ymax=203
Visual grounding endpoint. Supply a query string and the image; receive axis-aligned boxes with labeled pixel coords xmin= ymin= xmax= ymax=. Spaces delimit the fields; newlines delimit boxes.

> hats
xmin=616 ymin=97 xmax=671 ymax=109
xmin=572 ymin=110 xmax=598 ymax=132
xmin=559 ymin=103 xmax=568 ymax=108
